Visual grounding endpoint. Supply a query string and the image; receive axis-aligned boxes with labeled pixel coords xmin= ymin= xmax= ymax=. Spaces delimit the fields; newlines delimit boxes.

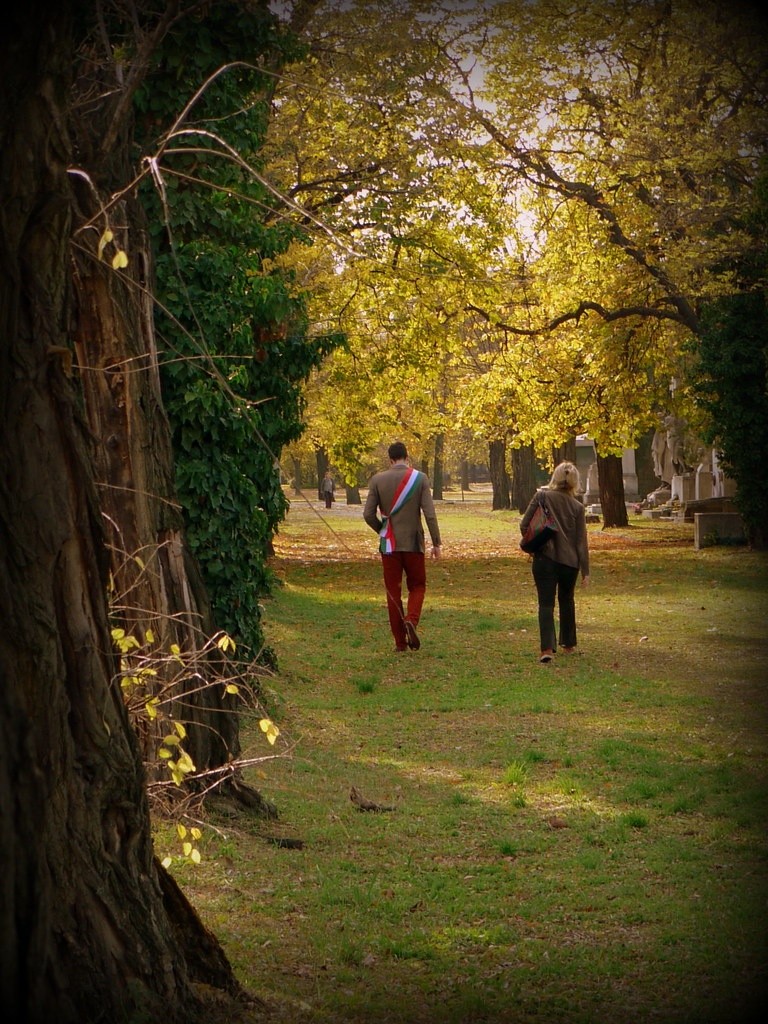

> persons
xmin=320 ymin=472 xmax=336 ymax=509
xmin=362 ymin=441 xmax=442 ymax=653
xmin=519 ymin=461 xmax=590 ymax=663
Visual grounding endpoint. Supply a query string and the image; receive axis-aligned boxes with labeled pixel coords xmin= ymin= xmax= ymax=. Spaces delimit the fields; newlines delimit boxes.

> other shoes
xmin=539 ymin=651 xmax=553 ymax=662
xmin=405 ymin=621 xmax=421 ymax=650
xmin=563 ymin=647 xmax=574 ymax=655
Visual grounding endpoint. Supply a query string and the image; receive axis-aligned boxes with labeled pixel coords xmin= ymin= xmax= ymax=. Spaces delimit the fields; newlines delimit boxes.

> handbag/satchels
xmin=520 ymin=502 xmax=559 ymax=554
